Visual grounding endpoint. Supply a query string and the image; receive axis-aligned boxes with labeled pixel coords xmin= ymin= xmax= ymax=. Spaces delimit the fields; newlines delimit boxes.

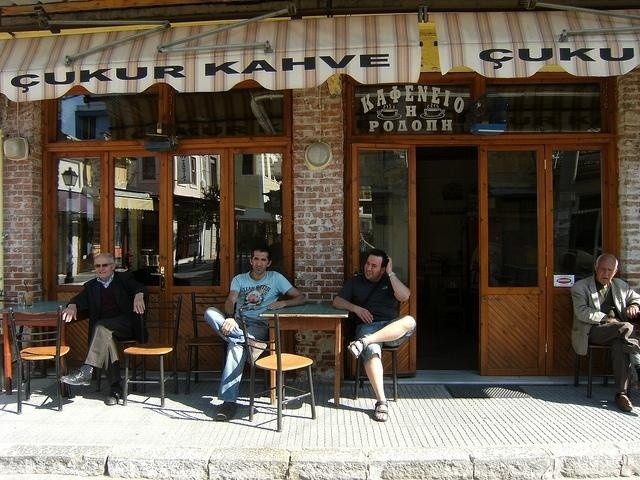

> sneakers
xmin=215 ymin=400 xmax=239 ymax=421
xmin=104 ymin=385 xmax=125 ymax=405
xmin=614 ymin=392 xmax=634 ymax=413
xmin=59 ymin=363 xmax=95 ymax=387
xmin=247 ymin=338 xmax=267 ymax=364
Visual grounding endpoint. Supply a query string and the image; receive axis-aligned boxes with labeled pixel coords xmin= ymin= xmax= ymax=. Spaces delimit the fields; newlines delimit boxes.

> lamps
xmin=304 ymin=140 xmax=334 ymax=171
xmin=3 ymin=136 xmax=29 ymax=161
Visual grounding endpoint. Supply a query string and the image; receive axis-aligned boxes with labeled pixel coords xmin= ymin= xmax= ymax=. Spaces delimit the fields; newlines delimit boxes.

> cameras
xmin=352 ymin=306 xmax=371 ymax=325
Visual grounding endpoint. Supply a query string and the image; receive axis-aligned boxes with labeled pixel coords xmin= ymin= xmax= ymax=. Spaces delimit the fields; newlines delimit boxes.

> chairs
xmin=570 ymin=298 xmax=614 ymax=398
xmin=355 ymin=302 xmax=401 ymax=402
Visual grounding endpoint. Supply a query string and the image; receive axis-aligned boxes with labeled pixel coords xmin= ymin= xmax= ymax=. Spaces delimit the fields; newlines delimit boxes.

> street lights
xmin=61 ymin=166 xmax=79 ymax=283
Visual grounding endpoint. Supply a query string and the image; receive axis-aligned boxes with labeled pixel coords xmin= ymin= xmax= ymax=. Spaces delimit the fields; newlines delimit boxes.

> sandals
xmin=373 ymin=400 xmax=390 ymax=422
xmin=347 ymin=339 xmax=367 ymax=360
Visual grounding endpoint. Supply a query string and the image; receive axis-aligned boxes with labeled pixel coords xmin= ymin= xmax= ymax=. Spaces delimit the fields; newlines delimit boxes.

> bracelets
xmin=388 ymin=272 xmax=397 ymax=279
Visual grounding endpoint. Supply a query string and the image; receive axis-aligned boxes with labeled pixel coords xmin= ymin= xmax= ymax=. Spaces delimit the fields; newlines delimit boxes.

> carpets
xmin=443 ymin=383 xmax=534 ymax=399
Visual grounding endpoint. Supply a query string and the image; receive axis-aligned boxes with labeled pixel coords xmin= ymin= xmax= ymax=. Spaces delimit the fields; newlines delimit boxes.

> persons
xmin=203 ymin=246 xmax=306 ymax=423
xmin=58 ymin=252 xmax=146 ymax=405
xmin=334 ymin=249 xmax=418 ymax=421
xmin=570 ymin=253 xmax=639 ymax=412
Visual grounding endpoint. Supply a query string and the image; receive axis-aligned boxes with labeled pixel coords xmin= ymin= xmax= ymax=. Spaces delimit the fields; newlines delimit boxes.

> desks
xmin=259 ymin=302 xmax=351 ymax=407
xmin=2 ymin=299 xmax=70 ymax=398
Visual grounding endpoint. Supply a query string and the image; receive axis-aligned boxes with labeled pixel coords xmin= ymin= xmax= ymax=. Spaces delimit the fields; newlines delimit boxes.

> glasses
xmin=93 ymin=261 xmax=114 ymax=268
